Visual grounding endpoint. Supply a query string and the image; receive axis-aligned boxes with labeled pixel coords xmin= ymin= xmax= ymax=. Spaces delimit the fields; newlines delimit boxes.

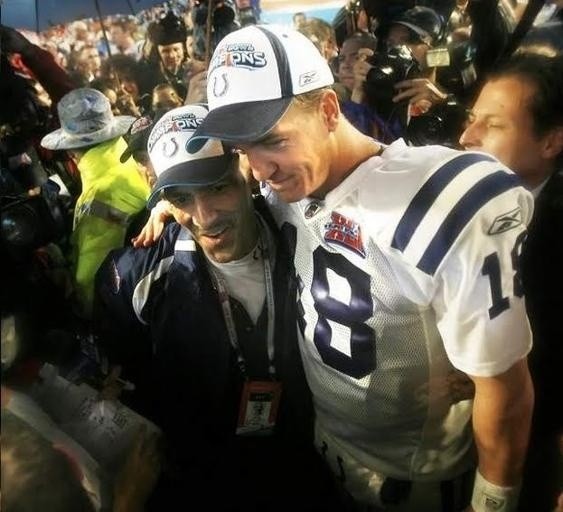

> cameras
xmin=0 ymin=198 xmax=42 ymax=251
xmin=192 ymin=0 xmax=236 ymax=26
xmin=406 ymin=93 xmax=468 ymax=146
xmin=362 ymin=48 xmax=422 ymax=101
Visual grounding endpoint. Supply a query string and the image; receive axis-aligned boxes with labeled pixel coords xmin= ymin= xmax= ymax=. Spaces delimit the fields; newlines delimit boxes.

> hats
xmin=185 ymin=23 xmax=335 ymax=156
xmin=38 ymin=85 xmax=137 ymax=151
xmin=389 ymin=7 xmax=446 ymax=49
xmin=116 ymin=109 xmax=167 ymax=164
xmin=143 ymin=104 xmax=237 ymax=212
